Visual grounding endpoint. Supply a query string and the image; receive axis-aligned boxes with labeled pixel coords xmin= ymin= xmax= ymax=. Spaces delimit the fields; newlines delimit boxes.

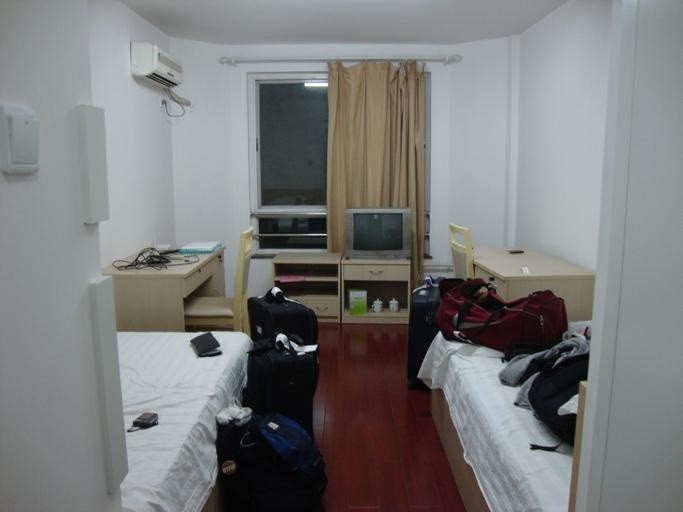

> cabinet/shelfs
xmin=342 ymin=258 xmax=411 ymax=324
xmin=271 ymin=251 xmax=341 ymax=323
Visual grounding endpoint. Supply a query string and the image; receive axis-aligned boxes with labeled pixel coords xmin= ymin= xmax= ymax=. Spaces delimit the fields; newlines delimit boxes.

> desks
xmin=102 ymin=243 xmax=226 ymax=332
xmin=474 ymin=246 xmax=595 ymax=320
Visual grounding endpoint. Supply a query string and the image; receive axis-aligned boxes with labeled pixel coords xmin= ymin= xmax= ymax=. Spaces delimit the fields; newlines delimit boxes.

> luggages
xmin=247 ymin=332 xmax=314 ymax=442
xmin=248 ymin=287 xmax=319 ymax=395
xmin=217 ymin=413 xmax=328 ymax=511
xmin=409 ymin=275 xmax=446 ymax=390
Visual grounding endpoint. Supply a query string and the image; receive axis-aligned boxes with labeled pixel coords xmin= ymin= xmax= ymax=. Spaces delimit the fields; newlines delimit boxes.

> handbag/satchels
xmin=529 ymin=354 xmax=589 ymax=446
xmin=436 ymin=278 xmax=567 ymax=355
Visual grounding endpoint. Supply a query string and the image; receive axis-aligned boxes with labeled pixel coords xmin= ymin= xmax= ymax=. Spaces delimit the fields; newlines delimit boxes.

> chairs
xmin=448 ymin=223 xmax=473 ymax=280
xmin=185 ymin=227 xmax=253 ymax=337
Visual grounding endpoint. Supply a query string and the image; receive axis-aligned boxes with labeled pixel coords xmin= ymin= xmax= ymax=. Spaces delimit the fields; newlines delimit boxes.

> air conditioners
xmin=129 ymin=40 xmax=191 ymax=106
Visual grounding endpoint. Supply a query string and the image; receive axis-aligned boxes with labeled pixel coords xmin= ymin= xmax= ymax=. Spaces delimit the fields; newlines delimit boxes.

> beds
xmin=415 ymin=319 xmax=590 ymax=510
xmin=117 ymin=332 xmax=254 ymax=512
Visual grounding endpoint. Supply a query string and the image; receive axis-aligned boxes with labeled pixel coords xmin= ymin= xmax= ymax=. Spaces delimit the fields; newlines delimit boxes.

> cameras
xmin=132 ymin=409 xmax=158 ymax=427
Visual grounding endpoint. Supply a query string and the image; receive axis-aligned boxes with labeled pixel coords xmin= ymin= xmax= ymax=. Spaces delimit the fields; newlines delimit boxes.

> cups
xmin=389 ymin=298 xmax=399 ymax=312
xmin=372 ymin=298 xmax=383 ymax=312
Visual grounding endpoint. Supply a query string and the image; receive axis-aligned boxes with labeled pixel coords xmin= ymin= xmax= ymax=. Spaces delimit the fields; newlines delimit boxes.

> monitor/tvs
xmin=343 ymin=207 xmax=412 ymax=262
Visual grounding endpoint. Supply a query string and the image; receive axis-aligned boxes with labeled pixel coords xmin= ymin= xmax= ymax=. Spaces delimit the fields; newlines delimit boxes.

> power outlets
xmin=160 ymin=95 xmax=169 ymax=107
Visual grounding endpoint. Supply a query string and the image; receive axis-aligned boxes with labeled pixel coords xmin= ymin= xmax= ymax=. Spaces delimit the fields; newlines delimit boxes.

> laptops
xmin=180 ymin=241 xmax=222 ymax=253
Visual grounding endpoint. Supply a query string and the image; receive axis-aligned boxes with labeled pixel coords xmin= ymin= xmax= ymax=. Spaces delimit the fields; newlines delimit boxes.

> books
xmin=190 ymin=333 xmax=222 ymax=357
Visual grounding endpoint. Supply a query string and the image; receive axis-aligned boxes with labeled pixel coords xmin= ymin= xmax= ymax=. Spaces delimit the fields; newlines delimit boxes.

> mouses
xmin=150 ymin=255 xmax=169 ymax=264
